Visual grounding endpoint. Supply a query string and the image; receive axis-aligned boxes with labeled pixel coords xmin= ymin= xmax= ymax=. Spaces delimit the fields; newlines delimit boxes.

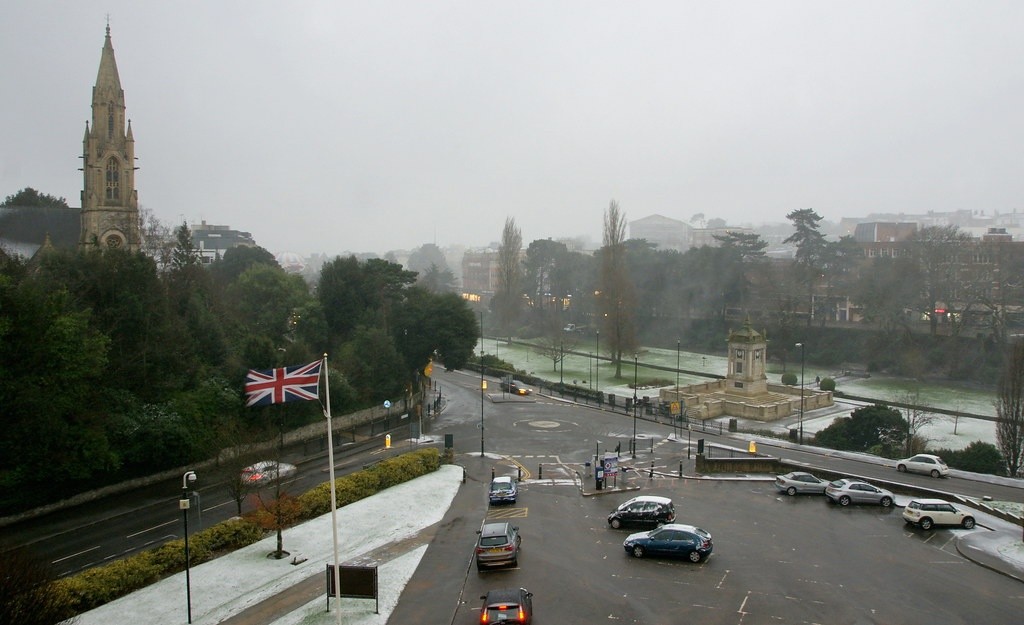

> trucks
xmin=563 ymin=324 xmax=588 ymax=332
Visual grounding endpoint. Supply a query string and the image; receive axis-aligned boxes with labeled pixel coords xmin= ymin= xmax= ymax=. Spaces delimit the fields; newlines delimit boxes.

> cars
xmin=895 ymin=453 xmax=949 ymax=478
xmin=241 ymin=461 xmax=298 ymax=488
xmin=500 ymin=380 xmax=533 ymax=395
xmin=774 ymin=471 xmax=831 ymax=496
xmin=902 ymin=499 xmax=976 ymax=530
xmin=607 ymin=496 xmax=679 ymax=528
xmin=824 ymin=477 xmax=896 ymax=507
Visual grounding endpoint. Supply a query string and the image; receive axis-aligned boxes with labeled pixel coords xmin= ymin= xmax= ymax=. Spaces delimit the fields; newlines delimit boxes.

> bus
xmin=725 ymin=308 xmax=809 ymax=324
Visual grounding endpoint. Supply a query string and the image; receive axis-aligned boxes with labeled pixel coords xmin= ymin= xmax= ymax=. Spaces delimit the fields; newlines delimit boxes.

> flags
xmin=244 ymin=359 xmax=322 ymax=409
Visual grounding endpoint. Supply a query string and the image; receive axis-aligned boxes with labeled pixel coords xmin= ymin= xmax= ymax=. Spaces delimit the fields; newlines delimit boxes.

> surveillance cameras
xmin=188 ymin=474 xmax=197 ymax=482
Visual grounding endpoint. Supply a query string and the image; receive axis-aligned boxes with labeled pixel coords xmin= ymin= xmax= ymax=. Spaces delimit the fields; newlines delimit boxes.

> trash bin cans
xmin=646 ymin=402 xmax=653 ymax=414
xmin=790 ymin=428 xmax=798 ymax=439
xmin=659 ymin=403 xmax=665 ymax=414
xmin=663 ymin=400 xmax=670 ymax=405
xmin=729 ymin=419 xmax=738 ymax=431
xmin=643 ymin=396 xmax=649 ymax=403
xmin=625 ymin=398 xmax=632 ymax=409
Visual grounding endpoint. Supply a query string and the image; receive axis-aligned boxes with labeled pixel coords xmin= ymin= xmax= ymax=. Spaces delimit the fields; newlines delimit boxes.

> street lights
xmin=794 ymin=343 xmax=804 ymax=445
xmin=479 ymin=350 xmax=485 ymax=455
xmin=595 ymin=328 xmax=600 ymax=391
xmin=180 ymin=469 xmax=197 ymax=624
xmin=632 ymin=352 xmax=638 ymax=458
xmin=677 ymin=336 xmax=681 ymax=402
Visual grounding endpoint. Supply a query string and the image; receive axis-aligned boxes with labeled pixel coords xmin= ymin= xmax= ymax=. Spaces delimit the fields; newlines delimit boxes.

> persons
xmin=815 ymin=375 xmax=820 ymax=385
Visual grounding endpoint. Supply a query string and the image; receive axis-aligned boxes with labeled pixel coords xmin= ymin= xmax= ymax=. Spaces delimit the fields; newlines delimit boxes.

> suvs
xmin=489 ymin=476 xmax=518 ymax=506
xmin=622 ymin=524 xmax=714 ymax=563
xmin=479 ymin=587 xmax=534 ymax=625
xmin=475 ymin=522 xmax=521 ymax=571
